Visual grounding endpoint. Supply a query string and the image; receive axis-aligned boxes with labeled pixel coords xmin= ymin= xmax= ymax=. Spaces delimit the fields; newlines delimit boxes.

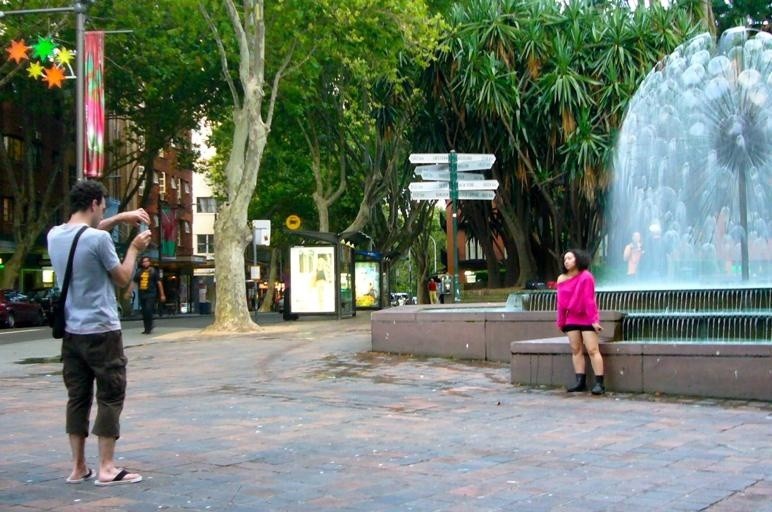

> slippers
xmin=94 ymin=468 xmax=143 ymax=486
xmin=66 ymin=469 xmax=95 ymax=483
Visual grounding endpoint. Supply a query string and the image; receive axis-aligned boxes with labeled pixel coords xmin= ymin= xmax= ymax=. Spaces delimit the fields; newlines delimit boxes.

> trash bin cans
xmin=200 ymin=303 xmax=209 ymax=314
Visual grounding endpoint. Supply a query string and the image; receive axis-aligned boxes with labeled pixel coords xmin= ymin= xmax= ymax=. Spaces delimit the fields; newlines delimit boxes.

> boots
xmin=567 ymin=373 xmax=587 ymax=392
xmin=591 ymin=375 xmax=605 ymax=394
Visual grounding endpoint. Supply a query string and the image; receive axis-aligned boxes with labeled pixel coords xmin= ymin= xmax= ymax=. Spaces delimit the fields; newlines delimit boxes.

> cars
xmin=278 ymin=294 xmax=285 ymax=313
xmin=391 ymin=291 xmax=419 ymax=306
xmin=0 ymin=286 xmax=60 ymax=328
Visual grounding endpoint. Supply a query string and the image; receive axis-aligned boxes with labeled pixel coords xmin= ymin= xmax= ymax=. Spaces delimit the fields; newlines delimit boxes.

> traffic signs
xmin=407 ymin=151 xmax=498 ymax=200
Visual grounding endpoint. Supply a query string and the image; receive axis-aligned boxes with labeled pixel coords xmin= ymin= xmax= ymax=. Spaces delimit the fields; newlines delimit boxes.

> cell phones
xmin=139 ymin=221 xmax=149 ymax=234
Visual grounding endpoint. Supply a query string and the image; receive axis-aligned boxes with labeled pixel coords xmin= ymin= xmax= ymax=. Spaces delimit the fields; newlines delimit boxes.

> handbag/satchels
xmin=47 ymin=297 xmax=67 ymax=339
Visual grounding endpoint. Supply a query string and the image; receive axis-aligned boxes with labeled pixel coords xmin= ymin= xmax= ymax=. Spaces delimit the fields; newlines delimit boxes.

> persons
xmin=556 ymin=249 xmax=605 ymax=395
xmin=427 ymin=279 xmax=439 ymax=304
xmin=123 ymin=256 xmax=165 ymax=334
xmin=622 ymin=220 xmax=666 ymax=281
xmin=47 ymin=181 xmax=152 ymax=487
